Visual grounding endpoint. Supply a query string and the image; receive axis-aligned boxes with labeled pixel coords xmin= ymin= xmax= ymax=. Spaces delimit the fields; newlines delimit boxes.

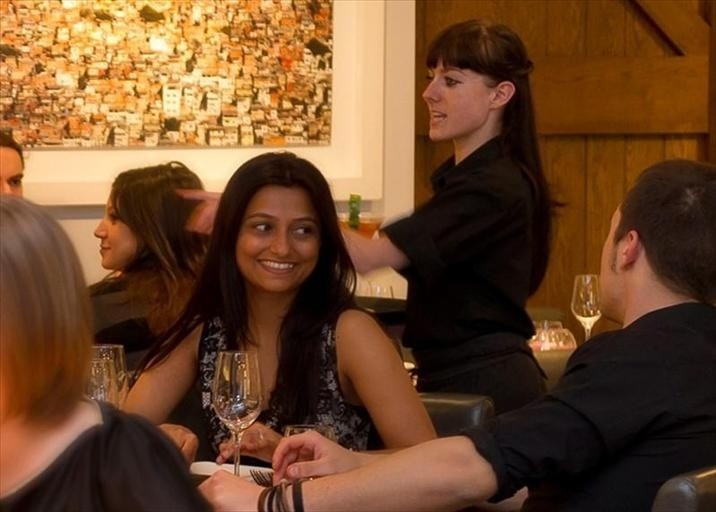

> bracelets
xmin=256 ymin=477 xmax=308 ymax=512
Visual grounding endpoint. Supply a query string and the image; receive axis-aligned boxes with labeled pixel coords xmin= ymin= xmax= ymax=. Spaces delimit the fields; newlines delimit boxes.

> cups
xmin=80 ymin=341 xmax=129 ymax=413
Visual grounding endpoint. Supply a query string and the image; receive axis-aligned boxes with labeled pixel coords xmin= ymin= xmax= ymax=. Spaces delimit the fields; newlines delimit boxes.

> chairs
xmin=652 ymin=466 xmax=716 ymax=512
xmin=418 ymin=391 xmax=496 ymax=439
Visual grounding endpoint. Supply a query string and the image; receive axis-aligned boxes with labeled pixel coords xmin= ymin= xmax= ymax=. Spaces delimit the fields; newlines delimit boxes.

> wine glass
xmin=208 ymin=350 xmax=261 ymax=478
xmin=569 ymin=275 xmax=604 ymax=340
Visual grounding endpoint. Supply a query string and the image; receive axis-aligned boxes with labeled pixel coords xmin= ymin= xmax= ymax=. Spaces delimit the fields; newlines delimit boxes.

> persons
xmin=176 ymin=19 xmax=571 ymax=414
xmin=78 ymin=161 xmax=216 ymax=371
xmin=1 ymin=131 xmax=26 ymax=198
xmin=195 ymin=160 xmax=715 ymax=512
xmin=121 ymin=149 xmax=438 ymax=464
xmin=1 ymin=194 xmax=214 ymax=511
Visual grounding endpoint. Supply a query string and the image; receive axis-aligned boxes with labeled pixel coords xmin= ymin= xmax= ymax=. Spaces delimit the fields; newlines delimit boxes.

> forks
xmin=247 ymin=467 xmax=276 ymax=487
